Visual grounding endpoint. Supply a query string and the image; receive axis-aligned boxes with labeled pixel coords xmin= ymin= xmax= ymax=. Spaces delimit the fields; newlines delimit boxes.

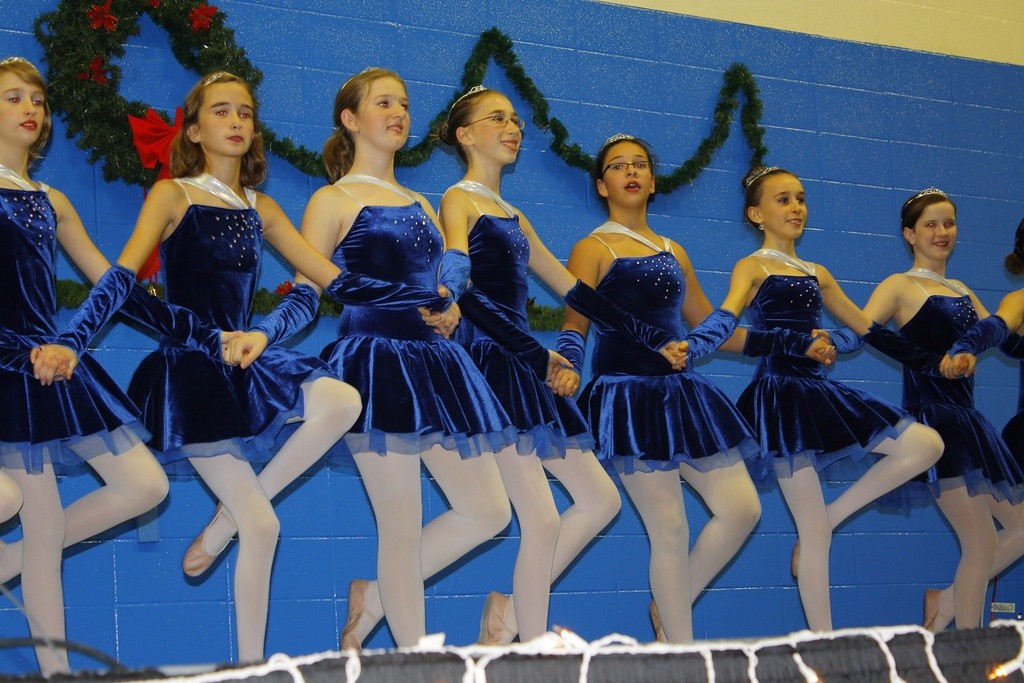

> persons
xmin=940 ymin=217 xmax=1024 ymax=475
xmin=665 ymin=166 xmax=976 ymax=633
xmin=552 ymin=134 xmax=836 ymax=643
xmin=222 ymin=66 xmax=574 ymax=653
xmin=30 ymin=72 xmax=462 ymax=664
xmin=0 ymin=58 xmax=247 ymax=679
xmin=418 ymin=85 xmax=687 ymax=645
xmin=811 ymin=185 xmax=1024 ymax=631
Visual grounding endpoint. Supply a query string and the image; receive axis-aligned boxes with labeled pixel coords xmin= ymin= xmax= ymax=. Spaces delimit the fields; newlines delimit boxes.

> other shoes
xmin=182 ymin=500 xmax=238 ymax=577
xmin=342 ymin=580 xmax=369 ymax=649
xmin=792 ymin=539 xmax=800 ymax=576
xmin=478 ymin=592 xmax=510 ymax=644
xmin=924 ymin=590 xmax=940 ymax=631
xmin=649 ymin=599 xmax=668 ymax=643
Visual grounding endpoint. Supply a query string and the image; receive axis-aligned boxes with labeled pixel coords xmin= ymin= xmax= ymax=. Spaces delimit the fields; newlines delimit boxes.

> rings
xmin=39 ymin=346 xmax=43 ymax=351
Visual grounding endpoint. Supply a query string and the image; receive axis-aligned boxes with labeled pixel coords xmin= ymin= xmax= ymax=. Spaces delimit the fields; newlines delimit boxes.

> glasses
xmin=602 ymin=160 xmax=651 ymax=179
xmin=463 ymin=114 xmax=526 ymax=130
